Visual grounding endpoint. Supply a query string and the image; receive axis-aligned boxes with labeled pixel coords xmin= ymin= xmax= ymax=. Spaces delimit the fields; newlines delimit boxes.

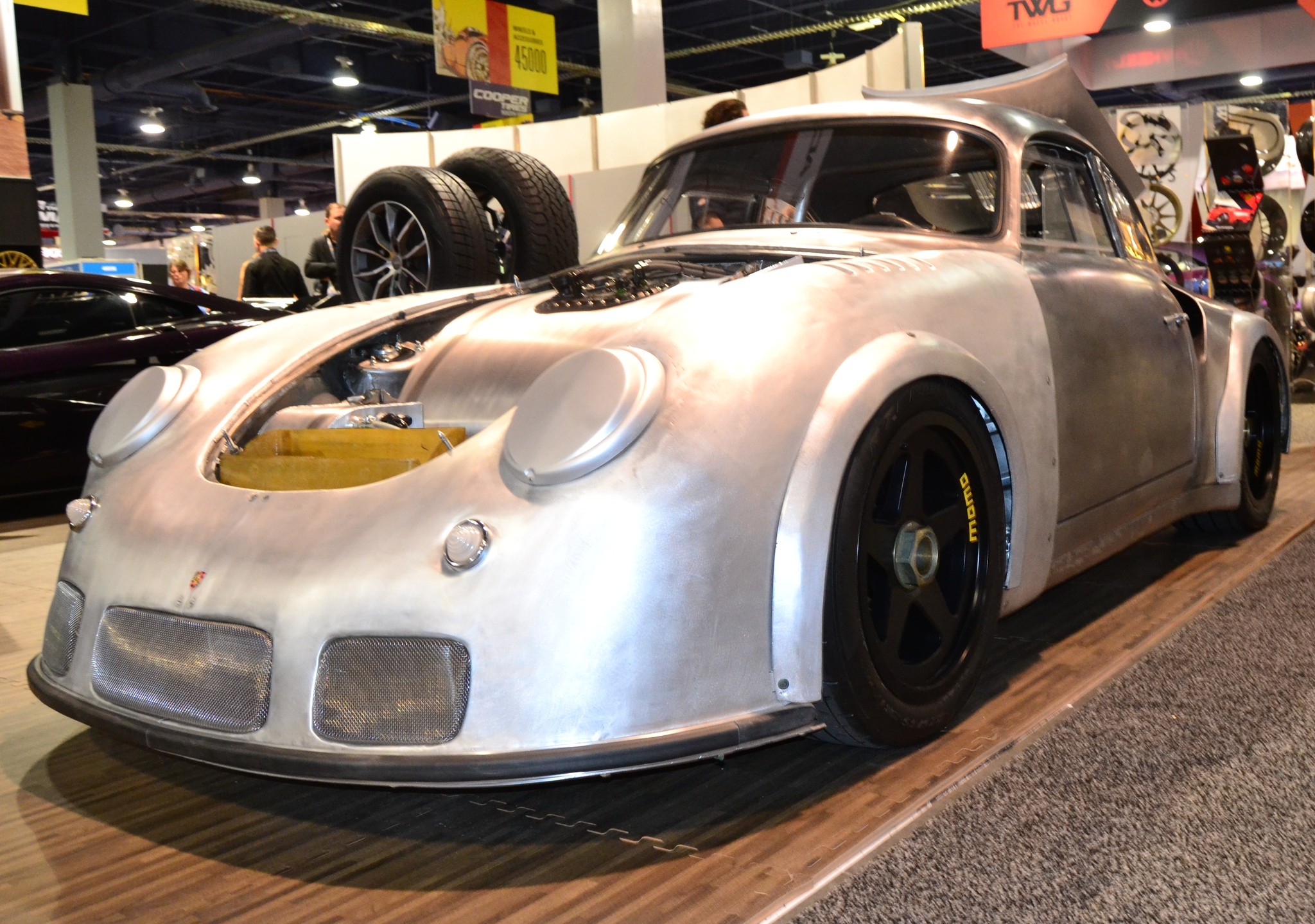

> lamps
xmin=331 ymin=55 xmax=359 ymax=87
xmin=139 ymin=107 xmax=165 ymax=134
xmin=294 ymin=199 xmax=310 ymax=216
xmin=189 ymin=220 xmax=205 ymax=232
xmin=114 ymin=188 xmax=133 ymax=208
xmin=241 ymin=164 xmax=261 ymax=184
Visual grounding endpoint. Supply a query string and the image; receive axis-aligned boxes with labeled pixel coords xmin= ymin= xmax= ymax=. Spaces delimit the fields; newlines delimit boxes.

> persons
xmin=169 ymin=257 xmax=214 ymax=315
xmin=687 ymin=97 xmax=765 ymax=231
xmin=237 ymin=225 xmax=310 ymax=302
xmin=303 ymin=202 xmax=350 ymax=295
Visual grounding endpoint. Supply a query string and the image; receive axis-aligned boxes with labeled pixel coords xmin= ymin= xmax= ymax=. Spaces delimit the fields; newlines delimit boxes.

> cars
xmin=21 ymin=88 xmax=1294 ymax=804
xmin=0 ymin=267 xmax=304 ymax=501
